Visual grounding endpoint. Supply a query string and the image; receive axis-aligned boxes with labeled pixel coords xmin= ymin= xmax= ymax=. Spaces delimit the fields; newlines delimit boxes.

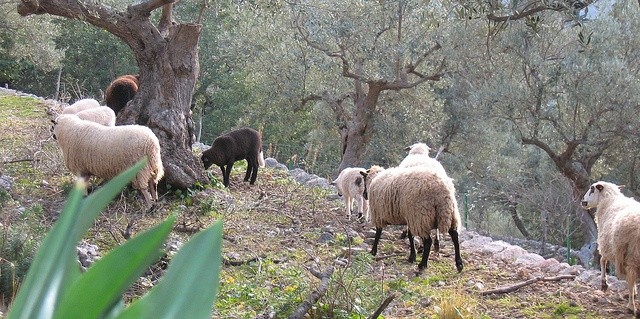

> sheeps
xmin=48 ymin=114 xmax=166 ymax=211
xmin=397 ymin=140 xmax=448 ymax=256
xmin=199 ymin=127 xmax=266 ymax=188
xmin=52 ymin=105 xmax=117 ymax=126
xmin=580 ymin=180 xmax=640 ymax=318
xmin=54 ymin=97 xmax=101 ymax=114
xmin=102 ymin=73 xmax=140 ymax=112
xmin=358 ymin=164 xmax=464 ymax=277
xmin=328 ymin=166 xmax=371 ymax=222
